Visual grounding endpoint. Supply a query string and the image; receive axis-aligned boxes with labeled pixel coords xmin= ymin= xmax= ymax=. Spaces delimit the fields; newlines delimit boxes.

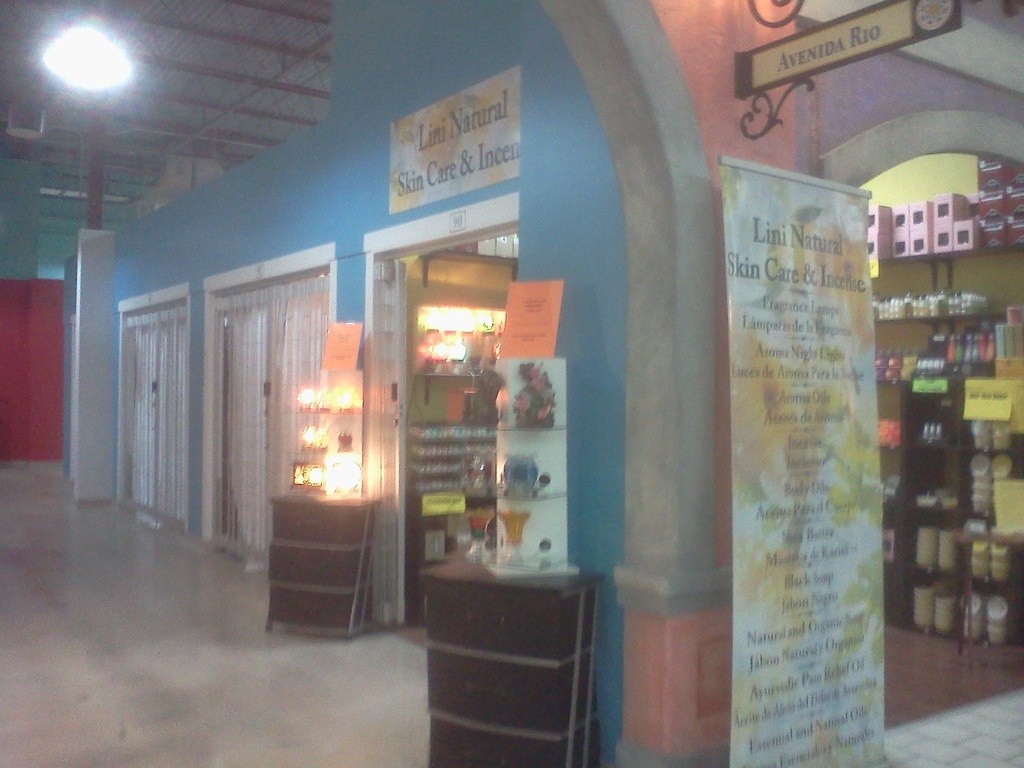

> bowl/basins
xmin=913 ymin=585 xmax=934 ymax=634
xmin=970 ymin=541 xmax=1013 ymax=582
xmin=971 ymin=478 xmax=993 ymax=512
xmin=962 ymin=619 xmax=1008 ymax=645
xmin=914 ymin=527 xmax=962 ymax=574
xmin=916 ymin=495 xmax=936 ymax=507
xmin=971 ymin=421 xmax=1008 ymax=450
xmin=935 ymin=594 xmax=956 ymax=634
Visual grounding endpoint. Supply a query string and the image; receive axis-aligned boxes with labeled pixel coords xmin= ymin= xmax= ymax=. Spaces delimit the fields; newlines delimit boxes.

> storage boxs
xmin=867 ymin=151 xmax=1024 ymax=262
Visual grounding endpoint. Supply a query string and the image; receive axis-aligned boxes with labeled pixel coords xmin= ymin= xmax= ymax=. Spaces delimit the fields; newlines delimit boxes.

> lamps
xmin=292 ymin=385 xmax=362 ymax=498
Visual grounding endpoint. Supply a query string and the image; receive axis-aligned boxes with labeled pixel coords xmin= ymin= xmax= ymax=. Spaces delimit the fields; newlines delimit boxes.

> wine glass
xmin=499 ymin=509 xmax=530 ymax=569
xmin=465 ymin=507 xmax=493 ymax=565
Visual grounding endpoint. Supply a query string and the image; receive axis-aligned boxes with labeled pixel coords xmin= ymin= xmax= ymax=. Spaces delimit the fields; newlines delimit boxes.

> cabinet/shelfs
xmin=868 ymin=242 xmax=1023 ymax=649
xmin=264 ymin=332 xmax=600 ymax=768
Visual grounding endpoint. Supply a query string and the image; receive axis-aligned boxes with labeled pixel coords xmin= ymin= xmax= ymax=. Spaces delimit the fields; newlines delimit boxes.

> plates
xmin=962 ymin=592 xmax=1009 ymax=621
xmin=970 ymin=455 xmax=1013 ymax=478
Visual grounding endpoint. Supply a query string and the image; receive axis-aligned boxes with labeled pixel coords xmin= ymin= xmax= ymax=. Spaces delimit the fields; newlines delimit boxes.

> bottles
xmin=503 ymin=451 xmax=538 ymax=499
xmin=873 ymin=287 xmax=990 ymax=318
xmin=922 ymin=420 xmax=944 ymax=444
xmin=876 ymin=325 xmax=996 ymax=379
